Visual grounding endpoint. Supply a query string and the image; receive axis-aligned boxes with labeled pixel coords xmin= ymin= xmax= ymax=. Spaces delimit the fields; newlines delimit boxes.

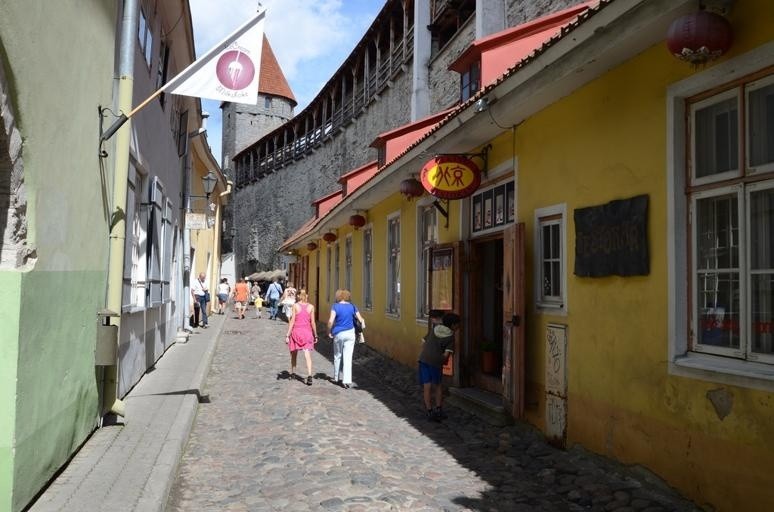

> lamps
xmin=221 ymin=227 xmax=236 ymax=239
xmin=186 ymin=165 xmax=218 ymax=201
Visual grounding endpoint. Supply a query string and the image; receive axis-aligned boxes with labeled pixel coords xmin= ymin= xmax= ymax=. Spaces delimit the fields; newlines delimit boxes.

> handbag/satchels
xmin=205 ymin=290 xmax=210 ymax=302
xmin=353 ymin=318 xmax=364 ymax=343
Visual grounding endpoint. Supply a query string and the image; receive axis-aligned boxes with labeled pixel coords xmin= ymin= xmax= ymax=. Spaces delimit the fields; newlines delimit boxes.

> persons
xmin=190 ymin=273 xmax=209 ymax=328
xmin=276 ymin=282 xmax=296 ymax=327
xmin=417 ymin=312 xmax=460 ymax=420
xmin=326 ymin=287 xmax=365 ymax=388
xmin=216 ymin=277 xmax=284 ymax=322
xmin=285 ymin=288 xmax=318 ymax=386
